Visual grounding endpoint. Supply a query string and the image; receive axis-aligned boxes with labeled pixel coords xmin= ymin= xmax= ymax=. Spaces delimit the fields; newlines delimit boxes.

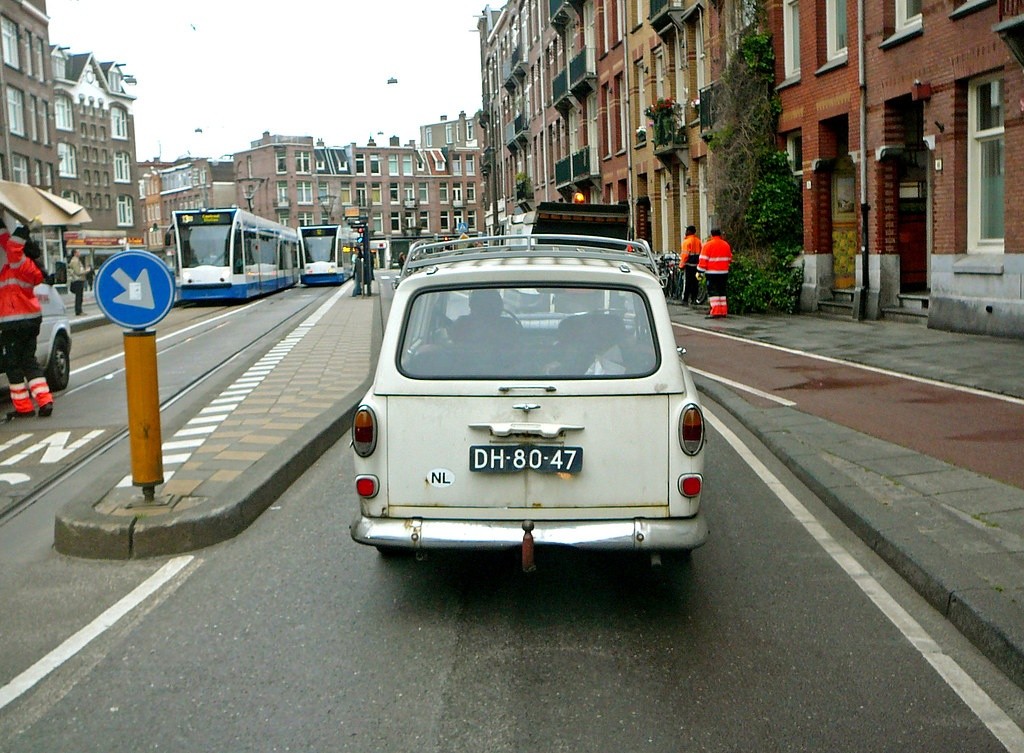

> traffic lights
xmin=358 ymin=228 xmax=363 ymax=233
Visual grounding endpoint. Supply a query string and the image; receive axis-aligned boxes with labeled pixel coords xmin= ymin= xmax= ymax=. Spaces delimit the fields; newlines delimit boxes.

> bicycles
xmin=656 ymin=248 xmax=706 ymax=302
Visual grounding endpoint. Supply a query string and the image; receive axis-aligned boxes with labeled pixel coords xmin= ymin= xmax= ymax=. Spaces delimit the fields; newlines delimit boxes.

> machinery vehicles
xmin=504 ymin=203 xmax=630 ymax=252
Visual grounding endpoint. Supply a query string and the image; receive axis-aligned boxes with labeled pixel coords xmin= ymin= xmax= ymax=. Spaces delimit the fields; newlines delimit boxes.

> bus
xmin=165 ymin=208 xmax=300 ymax=300
xmin=295 ymin=224 xmax=359 ymax=283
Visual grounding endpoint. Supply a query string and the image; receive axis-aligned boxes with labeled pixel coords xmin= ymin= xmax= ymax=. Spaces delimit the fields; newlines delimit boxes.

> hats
xmin=684 ymin=226 xmax=697 ymax=233
xmin=710 ymin=229 xmax=721 ymax=234
xmin=25 ymin=236 xmax=40 ymax=258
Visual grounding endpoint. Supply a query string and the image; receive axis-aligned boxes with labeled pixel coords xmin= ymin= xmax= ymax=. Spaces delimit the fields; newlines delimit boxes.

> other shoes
xmin=76 ymin=312 xmax=88 ymax=316
xmin=39 ymin=402 xmax=54 ymax=417
xmin=689 ymin=300 xmax=696 ymax=304
xmin=7 ymin=409 xmax=36 ymax=420
xmin=680 ymin=298 xmax=688 ymax=306
xmin=705 ymin=314 xmax=727 ymax=319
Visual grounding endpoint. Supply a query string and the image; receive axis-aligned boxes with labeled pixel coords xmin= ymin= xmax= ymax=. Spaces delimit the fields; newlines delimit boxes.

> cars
xmin=352 ymin=230 xmax=710 ymax=593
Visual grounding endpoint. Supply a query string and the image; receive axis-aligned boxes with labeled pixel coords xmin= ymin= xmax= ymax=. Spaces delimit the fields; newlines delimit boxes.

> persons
xmin=0 ymin=213 xmax=55 ymax=424
xmin=67 ymin=247 xmax=93 ymax=316
xmin=677 ymin=225 xmax=703 ymax=309
xmin=397 ymin=251 xmax=406 ymax=272
xmin=694 ymin=224 xmax=738 ymax=321
xmin=86 ymin=263 xmax=98 ymax=291
xmin=347 ymin=245 xmax=366 ymax=298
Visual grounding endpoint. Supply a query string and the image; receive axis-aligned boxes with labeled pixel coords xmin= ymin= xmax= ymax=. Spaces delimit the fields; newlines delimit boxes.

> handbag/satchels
xmin=69 ymin=282 xmax=81 ymax=294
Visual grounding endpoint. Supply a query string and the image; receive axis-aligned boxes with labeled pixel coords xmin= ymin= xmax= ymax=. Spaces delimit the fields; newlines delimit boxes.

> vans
xmin=0 ymin=218 xmax=72 ymax=393
